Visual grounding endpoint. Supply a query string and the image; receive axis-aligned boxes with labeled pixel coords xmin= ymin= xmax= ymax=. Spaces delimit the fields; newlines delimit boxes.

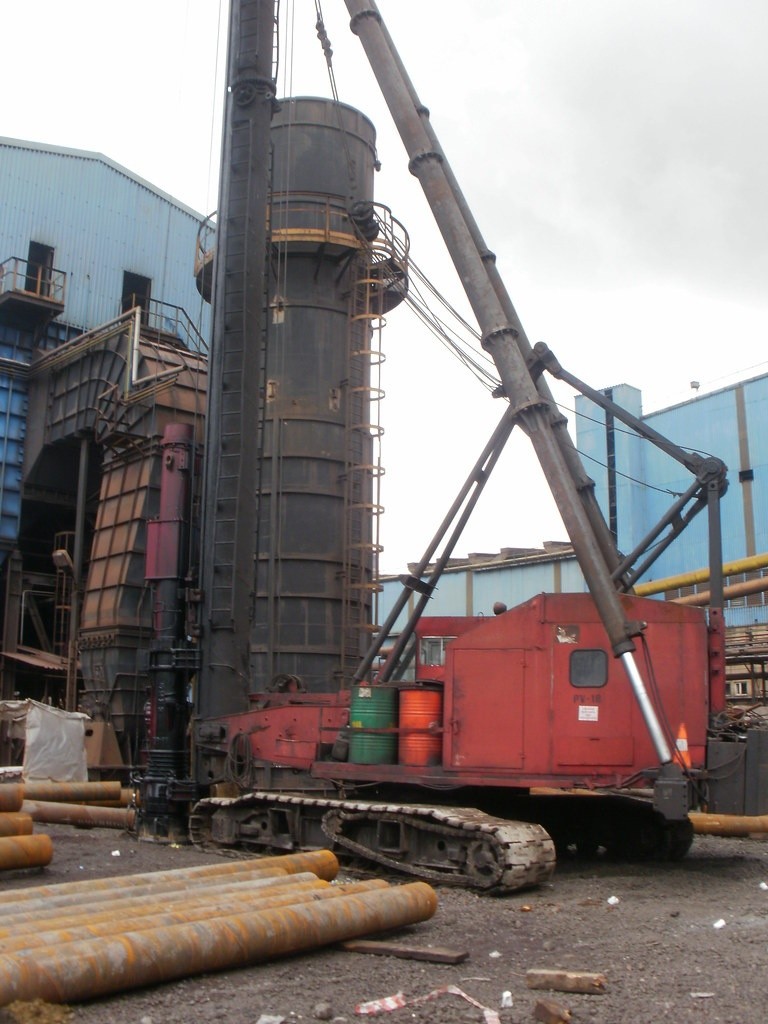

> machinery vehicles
xmin=189 ymin=0 xmax=730 ymax=894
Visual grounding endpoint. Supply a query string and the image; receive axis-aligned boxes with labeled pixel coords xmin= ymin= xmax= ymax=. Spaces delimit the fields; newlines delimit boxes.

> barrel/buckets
xmin=397 ymin=688 xmax=443 ymax=768
xmin=347 ymin=684 xmax=398 ymax=766
